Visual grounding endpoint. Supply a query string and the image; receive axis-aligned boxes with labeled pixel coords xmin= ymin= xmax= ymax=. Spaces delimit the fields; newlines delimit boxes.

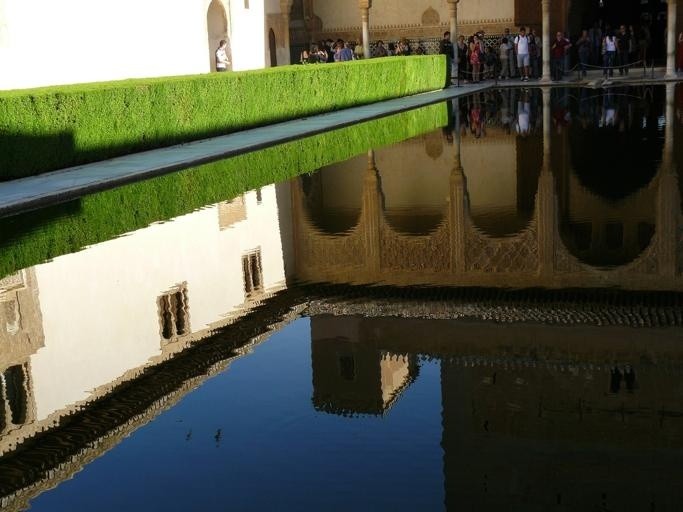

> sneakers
xmin=498 ymin=76 xmax=510 ymax=80
xmin=521 ymin=76 xmax=529 ymax=81
xmin=463 ymin=80 xmax=480 ymax=84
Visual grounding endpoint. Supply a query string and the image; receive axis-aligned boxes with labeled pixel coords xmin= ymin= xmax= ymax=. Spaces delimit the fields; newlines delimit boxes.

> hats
xmin=332 ymin=39 xmax=344 ymax=49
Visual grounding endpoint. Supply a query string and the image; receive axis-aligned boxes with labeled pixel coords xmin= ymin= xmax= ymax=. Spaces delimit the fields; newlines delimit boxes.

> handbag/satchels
xmin=602 ymin=41 xmax=606 ymax=54
xmin=478 ymin=51 xmax=488 ymax=65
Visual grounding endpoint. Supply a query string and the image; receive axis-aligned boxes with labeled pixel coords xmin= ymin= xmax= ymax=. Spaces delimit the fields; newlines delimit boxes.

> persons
xmin=438 ymin=26 xmax=543 ymax=87
xmin=551 ymin=22 xmax=631 ymax=81
xmin=215 ymin=40 xmax=231 ymax=72
xmin=441 ymin=88 xmax=660 ymax=144
xmin=299 ymin=38 xmax=413 ymax=65
